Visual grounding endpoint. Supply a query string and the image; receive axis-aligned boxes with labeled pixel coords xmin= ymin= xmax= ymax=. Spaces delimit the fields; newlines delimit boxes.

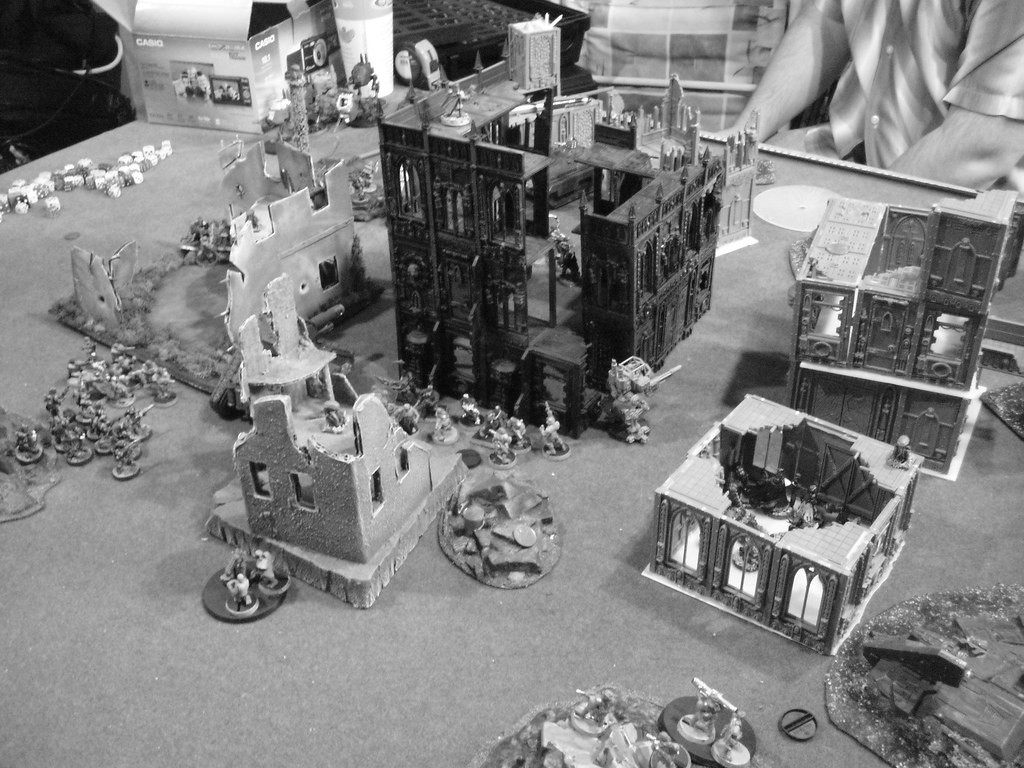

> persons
xmin=714 ymin=0 xmax=1024 ymax=190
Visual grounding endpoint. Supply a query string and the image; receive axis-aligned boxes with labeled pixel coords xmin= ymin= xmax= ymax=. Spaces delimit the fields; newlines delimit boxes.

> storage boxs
xmin=92 ymin=0 xmax=347 ymax=138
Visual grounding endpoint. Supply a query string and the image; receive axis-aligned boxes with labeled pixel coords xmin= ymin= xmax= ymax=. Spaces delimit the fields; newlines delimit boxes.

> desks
xmin=0 ymin=85 xmax=1024 ymax=768
